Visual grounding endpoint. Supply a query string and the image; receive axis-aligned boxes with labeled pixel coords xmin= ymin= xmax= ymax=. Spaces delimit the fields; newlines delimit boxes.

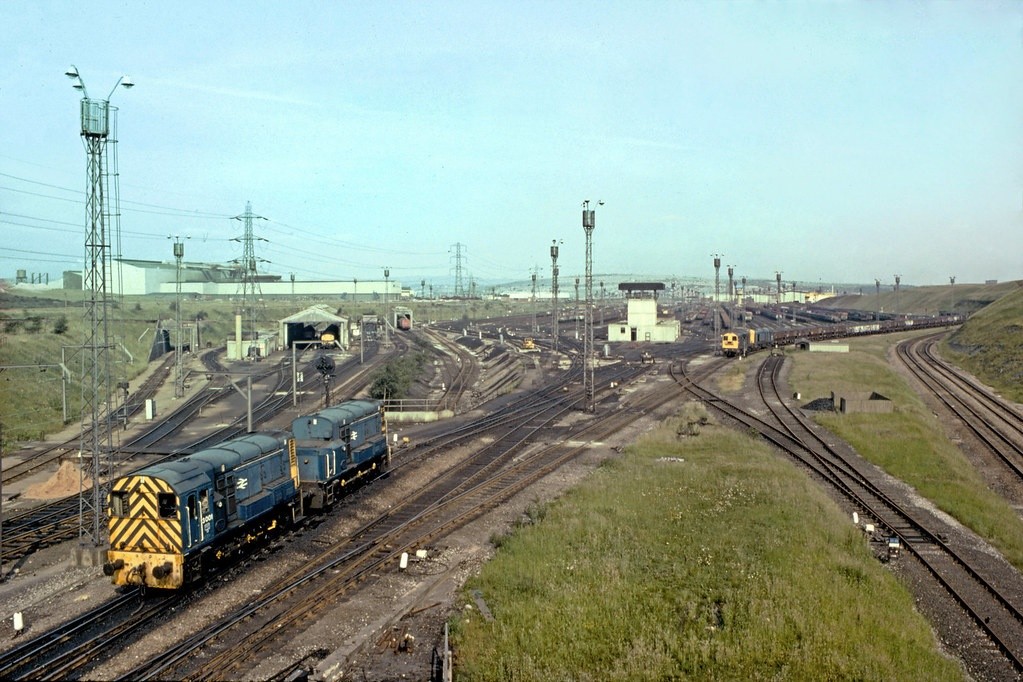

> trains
xmin=103 ymin=397 xmax=391 ymax=612
xmin=546 ymin=304 xmax=628 ymax=325
xmin=658 ymin=302 xmax=898 ymax=332
xmin=721 ymin=313 xmax=970 ymax=359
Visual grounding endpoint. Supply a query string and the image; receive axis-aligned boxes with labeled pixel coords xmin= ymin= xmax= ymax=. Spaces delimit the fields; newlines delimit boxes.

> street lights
xmin=288 ymin=271 xmax=297 ymax=294
xmin=380 ymin=266 xmax=392 ymax=351
xmin=600 ymin=282 xmax=603 ymax=301
xmin=550 ymin=238 xmax=564 ymax=378
xmin=874 ymin=278 xmax=883 ymax=313
xmin=894 ymin=274 xmax=903 ymax=319
xmin=580 ymin=198 xmax=604 ymax=414
xmin=574 ymin=278 xmax=580 ymax=342
xmin=710 ymin=252 xmax=725 ymax=356
xmin=353 ymin=278 xmax=358 ymax=293
xmin=949 ymin=276 xmax=956 ymax=316
xmin=727 ymin=264 xmax=737 ymax=332
xmin=768 ymin=271 xmax=797 ymax=304
xmin=492 ymin=287 xmax=495 ymax=301
xmin=671 ymin=281 xmax=705 ymax=304
xmin=740 ymin=275 xmax=749 ymax=330
xmin=531 ymin=274 xmax=537 ymax=343
xmin=65 ymin=64 xmax=135 ymax=568
xmin=421 ymin=279 xmax=425 ymax=322
xmin=166 ymin=233 xmax=191 ymax=402
xmin=472 ymin=282 xmax=476 ymax=327
xmin=429 ymin=284 xmax=433 ymax=322
